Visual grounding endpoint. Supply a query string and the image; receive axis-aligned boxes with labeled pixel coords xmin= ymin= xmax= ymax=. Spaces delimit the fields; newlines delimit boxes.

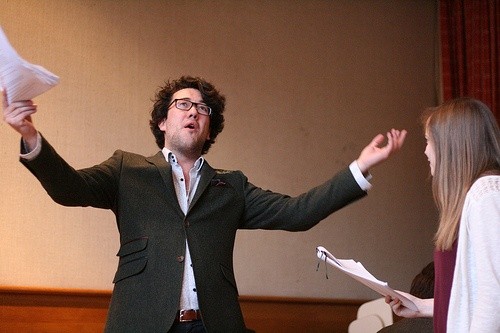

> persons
xmin=1 ymin=76 xmax=408 ymax=333
xmin=384 ymin=96 xmax=500 ymax=333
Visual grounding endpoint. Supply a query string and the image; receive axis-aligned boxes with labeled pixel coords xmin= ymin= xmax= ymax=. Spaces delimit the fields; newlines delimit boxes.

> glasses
xmin=167 ymin=99 xmax=212 ymax=116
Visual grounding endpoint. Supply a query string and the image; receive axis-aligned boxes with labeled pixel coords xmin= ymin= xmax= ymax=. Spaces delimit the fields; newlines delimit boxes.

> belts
xmin=174 ymin=309 xmax=202 ymax=322
